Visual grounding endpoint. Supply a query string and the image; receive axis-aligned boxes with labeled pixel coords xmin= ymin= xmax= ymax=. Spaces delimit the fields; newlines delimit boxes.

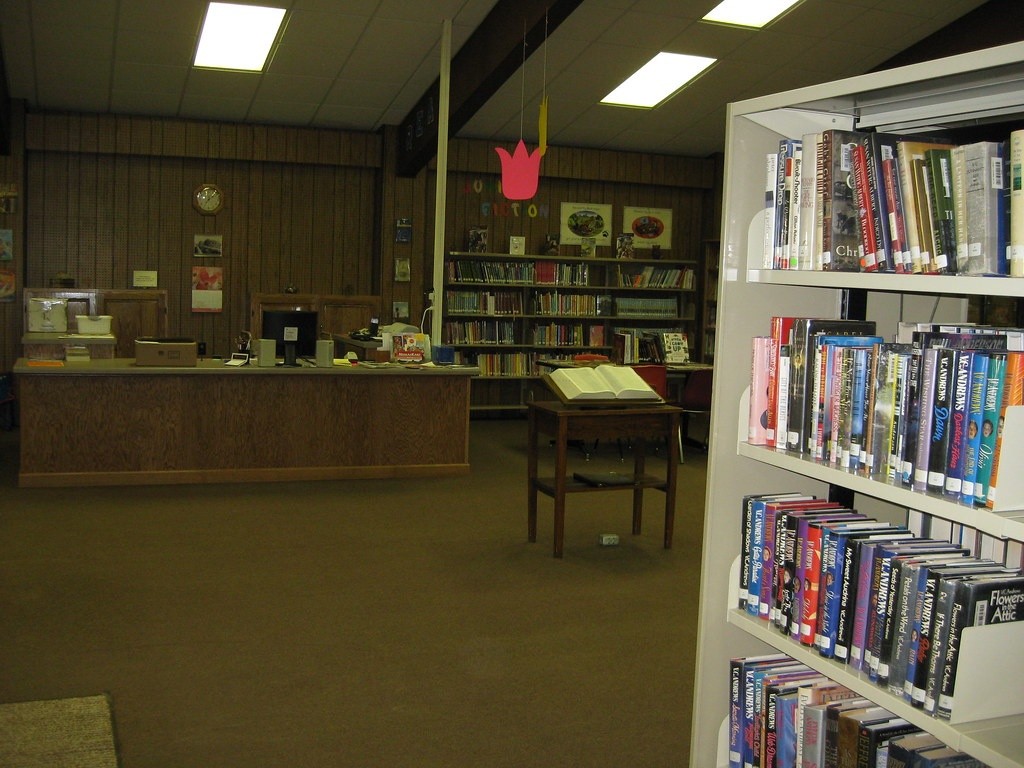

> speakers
xmin=315 ymin=340 xmax=334 ymax=368
xmin=257 ymin=339 xmax=276 ymax=367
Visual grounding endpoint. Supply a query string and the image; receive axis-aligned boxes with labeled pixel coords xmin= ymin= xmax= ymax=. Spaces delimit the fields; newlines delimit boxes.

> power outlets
xmin=432 ymin=291 xmax=439 ymax=310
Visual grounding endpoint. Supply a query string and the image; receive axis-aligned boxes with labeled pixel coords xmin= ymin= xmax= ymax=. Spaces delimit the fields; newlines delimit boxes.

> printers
xmin=134 ymin=336 xmax=198 ymax=368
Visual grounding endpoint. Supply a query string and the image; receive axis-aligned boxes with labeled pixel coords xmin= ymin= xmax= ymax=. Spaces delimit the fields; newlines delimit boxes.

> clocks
xmin=192 ymin=183 xmax=224 ymax=217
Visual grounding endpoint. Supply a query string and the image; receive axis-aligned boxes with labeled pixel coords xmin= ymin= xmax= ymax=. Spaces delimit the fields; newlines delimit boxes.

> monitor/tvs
xmin=261 ymin=310 xmax=317 ymax=368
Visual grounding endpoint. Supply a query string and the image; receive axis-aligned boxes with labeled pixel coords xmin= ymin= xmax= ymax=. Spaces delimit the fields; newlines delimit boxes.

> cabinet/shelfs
xmin=250 ymin=292 xmax=382 ymax=357
xmin=687 ymin=40 xmax=1024 ymax=768
xmin=699 ymin=238 xmax=720 ymax=365
xmin=535 ymin=358 xmax=713 ymax=464
xmin=22 ymin=287 xmax=169 ymax=359
xmin=442 ymin=249 xmax=700 ymax=411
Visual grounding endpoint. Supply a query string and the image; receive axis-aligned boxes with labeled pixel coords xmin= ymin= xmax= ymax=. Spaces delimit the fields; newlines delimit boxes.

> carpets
xmin=0 ymin=690 xmax=123 ymax=768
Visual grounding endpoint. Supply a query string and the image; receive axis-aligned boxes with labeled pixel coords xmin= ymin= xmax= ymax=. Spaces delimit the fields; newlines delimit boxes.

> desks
xmin=523 ymin=399 xmax=686 ymax=560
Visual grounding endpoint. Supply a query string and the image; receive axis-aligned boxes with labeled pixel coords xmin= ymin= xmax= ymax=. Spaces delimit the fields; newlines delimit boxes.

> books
xmin=749 ymin=315 xmax=1024 ymax=514
xmin=737 ymin=491 xmax=1024 ymax=721
xmin=445 ymin=259 xmax=694 ymax=290
xmin=762 ymin=129 xmax=1024 ymax=278
xmin=446 ymin=289 xmax=679 ymax=319
xmin=548 ymin=365 xmax=659 ymax=400
xmin=444 ymin=320 xmax=604 ymax=348
xmin=455 ymin=351 xmax=608 ymax=377
xmin=726 ymin=653 xmax=988 ymax=768
xmin=612 ymin=328 xmax=691 ymax=365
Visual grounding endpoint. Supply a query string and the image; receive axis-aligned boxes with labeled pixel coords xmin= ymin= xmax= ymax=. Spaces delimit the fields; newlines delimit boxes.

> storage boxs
xmin=75 ymin=315 xmax=114 ymax=336
xmin=133 ymin=339 xmax=197 ymax=367
